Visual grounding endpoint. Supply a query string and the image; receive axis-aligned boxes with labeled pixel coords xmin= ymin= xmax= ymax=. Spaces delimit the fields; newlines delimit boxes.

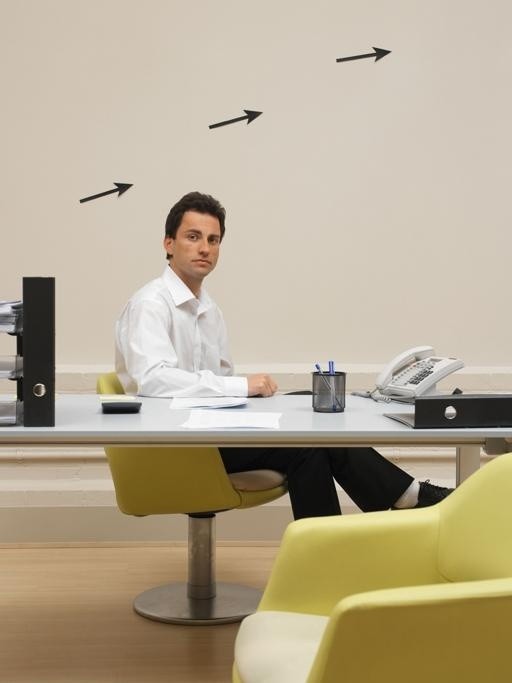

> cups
xmin=310 ymin=371 xmax=348 ymax=413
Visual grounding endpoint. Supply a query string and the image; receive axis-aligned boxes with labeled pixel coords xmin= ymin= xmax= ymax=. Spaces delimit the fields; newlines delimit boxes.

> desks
xmin=0 ymin=394 xmax=512 ymax=493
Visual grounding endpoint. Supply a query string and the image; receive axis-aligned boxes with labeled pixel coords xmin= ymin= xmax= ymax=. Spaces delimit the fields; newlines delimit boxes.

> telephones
xmin=376 ymin=346 xmax=464 ymax=404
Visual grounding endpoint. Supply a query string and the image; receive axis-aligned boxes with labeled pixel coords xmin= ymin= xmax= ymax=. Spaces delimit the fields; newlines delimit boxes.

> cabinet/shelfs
xmin=0 ymin=277 xmax=55 ymax=428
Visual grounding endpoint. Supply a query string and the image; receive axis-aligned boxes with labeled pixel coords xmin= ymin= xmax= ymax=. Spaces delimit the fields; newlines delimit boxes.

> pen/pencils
xmin=316 ymin=361 xmax=343 ymax=410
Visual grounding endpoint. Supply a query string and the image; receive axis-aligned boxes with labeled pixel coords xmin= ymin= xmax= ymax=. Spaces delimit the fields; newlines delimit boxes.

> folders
xmin=384 ymin=395 xmax=512 ymax=429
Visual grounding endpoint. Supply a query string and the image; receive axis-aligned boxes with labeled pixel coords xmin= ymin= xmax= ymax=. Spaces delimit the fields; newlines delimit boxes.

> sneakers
xmin=391 ymin=482 xmax=455 ymax=509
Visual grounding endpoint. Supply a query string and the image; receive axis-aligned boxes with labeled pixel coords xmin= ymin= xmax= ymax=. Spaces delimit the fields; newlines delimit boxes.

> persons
xmin=114 ymin=190 xmax=455 ymax=524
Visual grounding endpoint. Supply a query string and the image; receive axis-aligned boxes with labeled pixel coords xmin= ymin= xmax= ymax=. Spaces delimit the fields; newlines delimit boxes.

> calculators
xmin=98 ymin=396 xmax=142 ymax=414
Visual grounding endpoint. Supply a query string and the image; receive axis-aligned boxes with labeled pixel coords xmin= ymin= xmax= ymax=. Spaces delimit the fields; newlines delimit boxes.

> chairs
xmin=230 ymin=452 xmax=512 ymax=683
xmin=95 ymin=369 xmax=291 ymax=627
